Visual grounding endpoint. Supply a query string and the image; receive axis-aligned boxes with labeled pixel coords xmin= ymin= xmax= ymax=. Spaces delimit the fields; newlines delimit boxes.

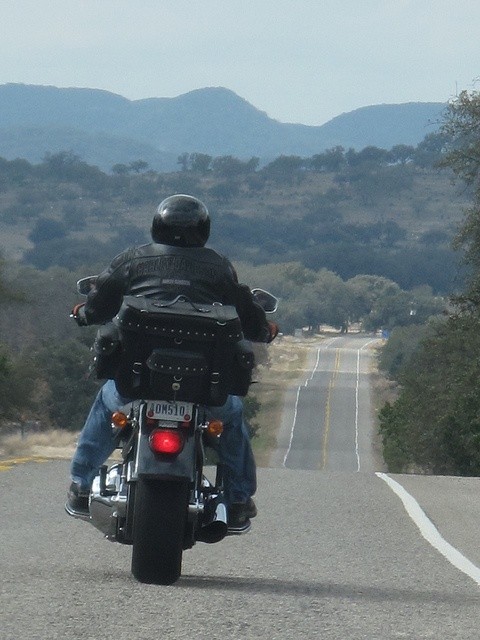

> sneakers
xmin=226 ymin=500 xmax=258 ymax=527
xmin=68 ymin=482 xmax=91 ymax=508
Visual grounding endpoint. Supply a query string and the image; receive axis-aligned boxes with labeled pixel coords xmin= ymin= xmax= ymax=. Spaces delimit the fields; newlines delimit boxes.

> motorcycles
xmin=76 ymin=276 xmax=279 ymax=584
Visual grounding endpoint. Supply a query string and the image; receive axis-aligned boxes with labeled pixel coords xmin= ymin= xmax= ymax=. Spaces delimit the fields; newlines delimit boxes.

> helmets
xmin=151 ymin=194 xmax=211 ymax=247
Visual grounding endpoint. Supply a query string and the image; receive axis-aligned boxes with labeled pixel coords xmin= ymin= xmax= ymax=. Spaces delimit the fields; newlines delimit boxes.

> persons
xmin=67 ymin=195 xmax=276 ymax=527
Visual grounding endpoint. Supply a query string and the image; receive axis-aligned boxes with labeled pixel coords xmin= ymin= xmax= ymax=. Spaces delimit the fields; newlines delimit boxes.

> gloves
xmin=71 ymin=303 xmax=88 ymax=327
xmin=266 ymin=320 xmax=278 ymax=343
